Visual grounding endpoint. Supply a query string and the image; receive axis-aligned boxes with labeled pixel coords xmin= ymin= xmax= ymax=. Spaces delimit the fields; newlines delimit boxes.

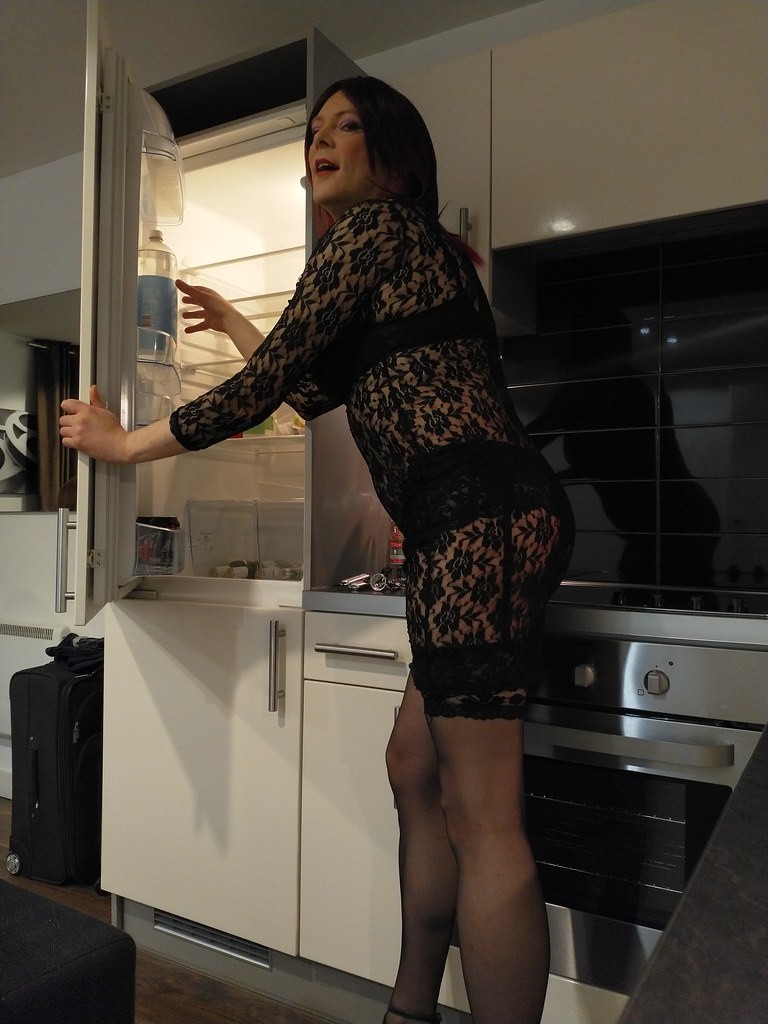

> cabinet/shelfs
xmin=298 ymin=610 xmax=413 ymax=988
xmin=102 ymin=589 xmax=314 ymax=963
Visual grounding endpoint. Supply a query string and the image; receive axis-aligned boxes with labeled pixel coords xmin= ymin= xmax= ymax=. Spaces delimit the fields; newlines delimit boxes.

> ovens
xmin=443 ymin=587 xmax=768 ymax=1024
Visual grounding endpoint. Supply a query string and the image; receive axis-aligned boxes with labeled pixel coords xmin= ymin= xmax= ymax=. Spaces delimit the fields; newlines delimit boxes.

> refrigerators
xmin=56 ymin=1 xmax=391 ymax=955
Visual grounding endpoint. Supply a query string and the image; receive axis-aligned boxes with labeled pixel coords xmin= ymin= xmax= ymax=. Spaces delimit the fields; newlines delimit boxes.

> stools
xmin=0 ymin=878 xmax=136 ymax=1024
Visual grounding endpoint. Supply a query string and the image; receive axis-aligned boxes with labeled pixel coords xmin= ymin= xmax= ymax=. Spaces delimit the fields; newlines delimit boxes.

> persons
xmin=57 ymin=75 xmax=574 ymax=1024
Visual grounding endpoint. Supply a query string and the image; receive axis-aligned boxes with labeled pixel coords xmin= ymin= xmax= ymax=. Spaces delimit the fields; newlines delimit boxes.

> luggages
xmin=4 ymin=632 xmax=104 ymax=886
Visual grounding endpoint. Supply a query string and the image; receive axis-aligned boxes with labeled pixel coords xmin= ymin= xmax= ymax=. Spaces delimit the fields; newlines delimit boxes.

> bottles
xmin=138 ymin=230 xmax=177 ymax=361
xmin=390 ymin=526 xmax=408 ymax=590
